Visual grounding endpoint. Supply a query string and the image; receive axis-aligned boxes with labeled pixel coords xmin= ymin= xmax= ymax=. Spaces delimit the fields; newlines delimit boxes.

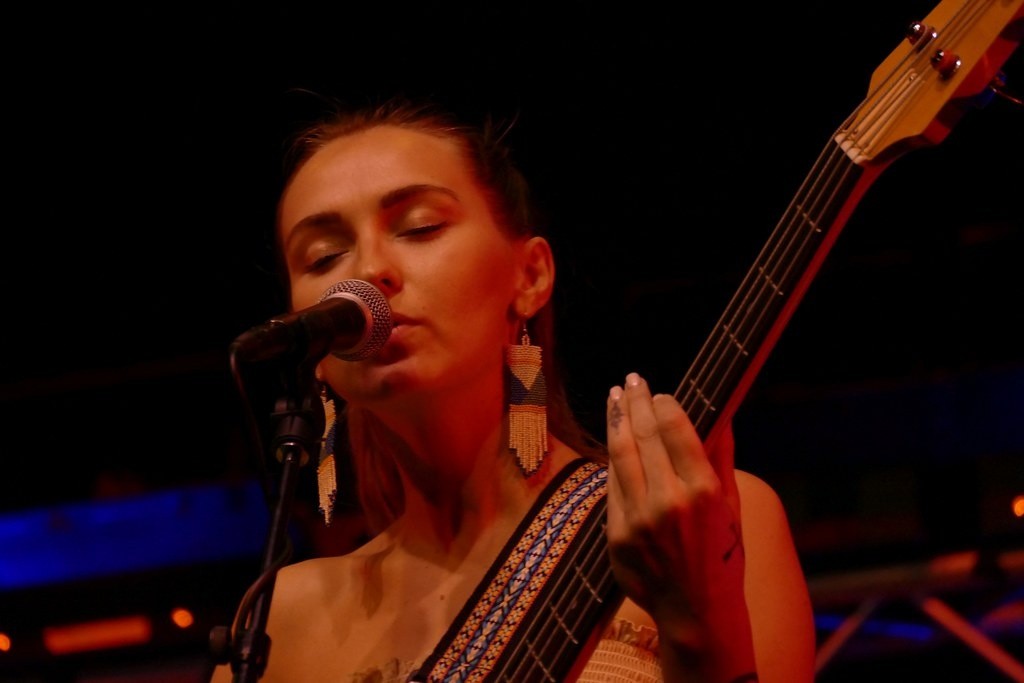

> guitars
xmin=479 ymin=0 xmax=1024 ymax=683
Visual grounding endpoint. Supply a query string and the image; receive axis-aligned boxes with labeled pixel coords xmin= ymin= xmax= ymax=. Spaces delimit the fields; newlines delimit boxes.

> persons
xmin=211 ymin=107 xmax=818 ymax=683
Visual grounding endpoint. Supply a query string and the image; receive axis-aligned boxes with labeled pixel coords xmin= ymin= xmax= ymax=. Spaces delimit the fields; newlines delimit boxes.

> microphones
xmin=231 ymin=278 xmax=393 ymax=384
